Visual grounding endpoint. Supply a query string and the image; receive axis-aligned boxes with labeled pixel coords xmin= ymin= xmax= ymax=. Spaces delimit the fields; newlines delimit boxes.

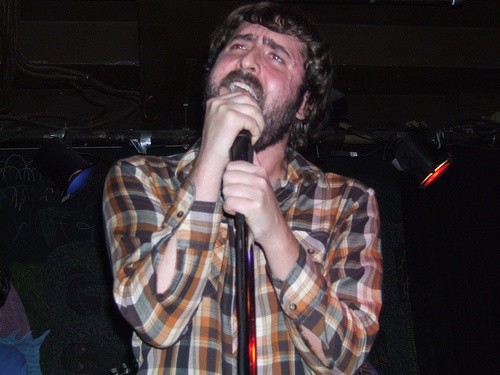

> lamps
xmin=387 ymin=126 xmax=454 ymax=190
xmin=34 ymin=137 xmax=99 ymax=202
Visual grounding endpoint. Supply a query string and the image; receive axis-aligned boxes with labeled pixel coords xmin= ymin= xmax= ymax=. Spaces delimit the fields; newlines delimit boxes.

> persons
xmin=101 ymin=2 xmax=383 ymax=375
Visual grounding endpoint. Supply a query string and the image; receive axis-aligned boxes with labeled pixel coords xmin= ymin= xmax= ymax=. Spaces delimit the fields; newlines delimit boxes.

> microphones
xmin=230 ymin=128 xmax=253 ymax=224
